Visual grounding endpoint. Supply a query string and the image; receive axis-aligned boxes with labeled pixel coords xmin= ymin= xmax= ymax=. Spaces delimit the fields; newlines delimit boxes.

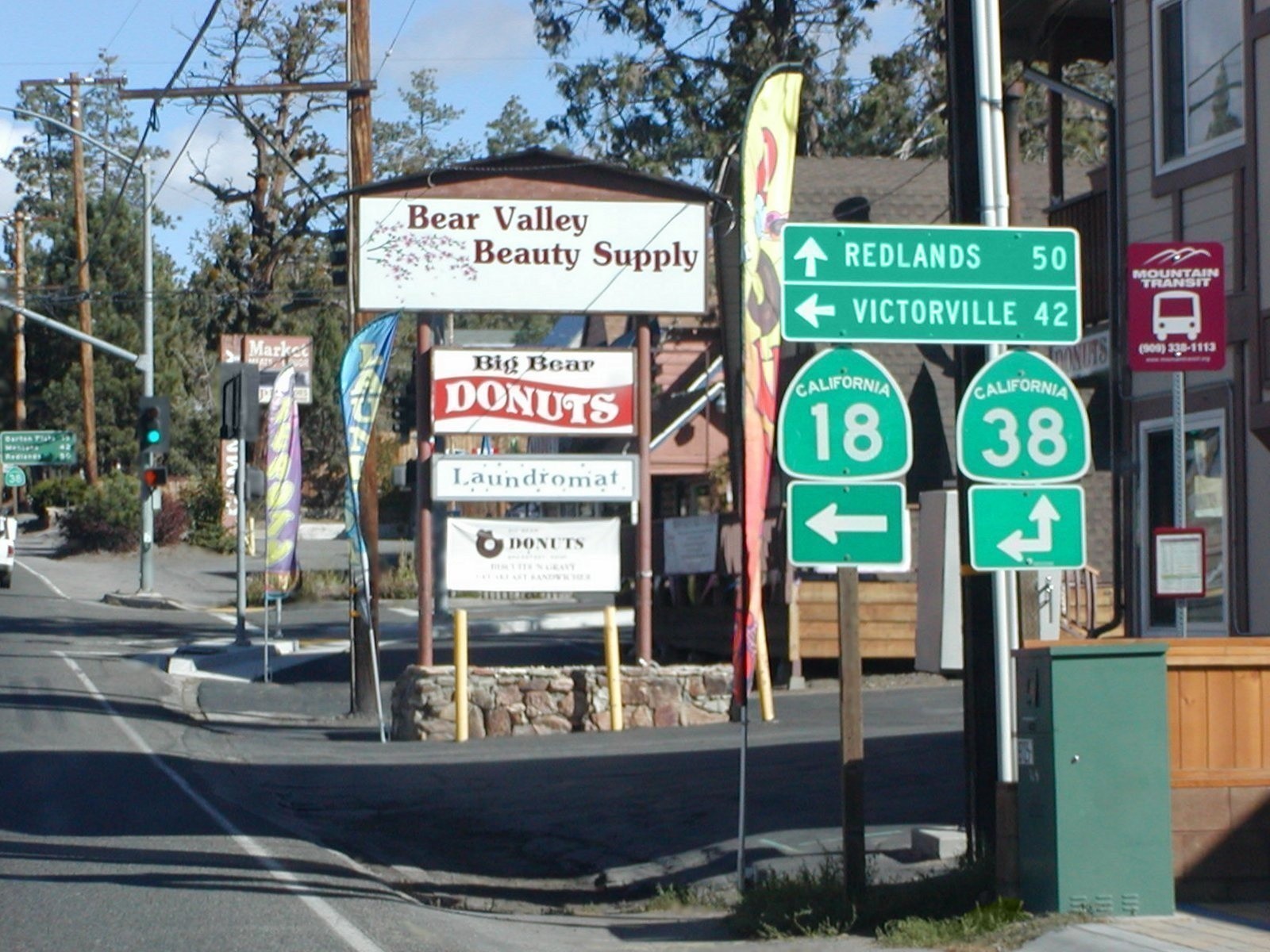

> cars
xmin=0 ymin=514 xmax=17 ymax=587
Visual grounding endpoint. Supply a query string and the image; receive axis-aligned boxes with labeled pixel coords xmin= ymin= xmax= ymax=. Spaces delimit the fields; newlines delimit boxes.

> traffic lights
xmin=139 ymin=395 xmax=173 ymax=454
xmin=327 ymin=226 xmax=352 ymax=286
xmin=142 ymin=468 xmax=171 ymax=487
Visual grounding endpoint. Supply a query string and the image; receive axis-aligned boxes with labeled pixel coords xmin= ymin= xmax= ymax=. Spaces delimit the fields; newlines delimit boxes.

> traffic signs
xmin=0 ymin=430 xmax=76 ymax=465
xmin=782 ymin=222 xmax=1083 ymax=282
xmin=782 ymin=282 xmax=1085 ymax=346
